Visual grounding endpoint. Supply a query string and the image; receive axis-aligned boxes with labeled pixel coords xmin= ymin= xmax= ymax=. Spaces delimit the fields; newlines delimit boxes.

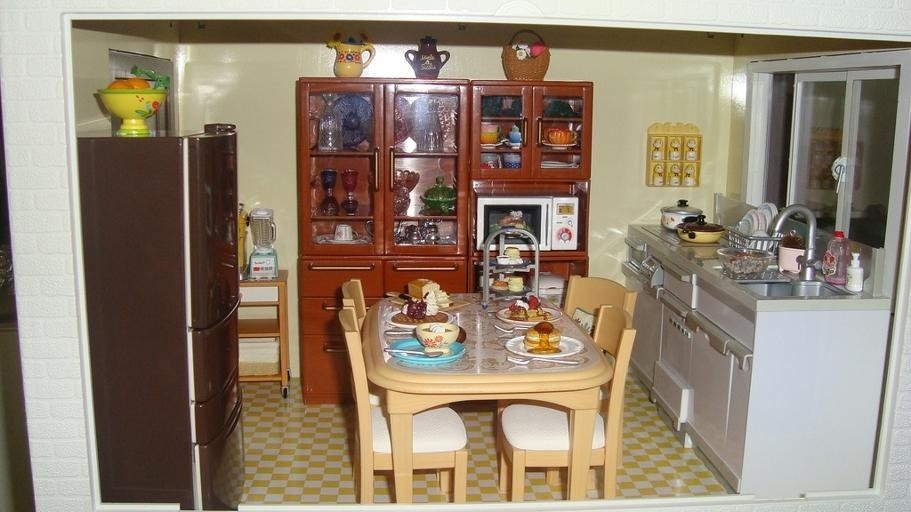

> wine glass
xmin=319 ymin=94 xmax=346 ymax=151
xmin=415 ymin=95 xmax=448 ymax=153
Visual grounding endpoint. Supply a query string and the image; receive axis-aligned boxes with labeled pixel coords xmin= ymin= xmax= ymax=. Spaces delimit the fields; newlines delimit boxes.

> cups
xmin=652 ymin=176 xmax=696 ymax=188
xmin=481 ymin=120 xmax=501 ymax=144
xmin=334 ymin=225 xmax=360 ymax=242
xmin=652 ymin=150 xmax=695 ymax=162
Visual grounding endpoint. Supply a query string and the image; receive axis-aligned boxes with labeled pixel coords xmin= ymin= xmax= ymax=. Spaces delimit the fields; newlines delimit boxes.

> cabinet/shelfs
xmin=685 ymin=264 xmax=892 ymax=498
xmin=647 ymin=133 xmax=702 ymax=190
xmin=619 ymin=224 xmax=664 ymax=402
xmin=469 ymin=79 xmax=593 ymax=312
xmin=649 ymin=251 xmax=694 ymax=450
xmin=238 ymin=269 xmax=290 ymax=399
xmin=298 ymin=76 xmax=468 ymax=405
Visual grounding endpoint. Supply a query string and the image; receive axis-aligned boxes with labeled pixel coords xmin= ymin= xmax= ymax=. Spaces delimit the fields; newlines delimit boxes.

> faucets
xmin=772 ymin=204 xmax=823 ymax=281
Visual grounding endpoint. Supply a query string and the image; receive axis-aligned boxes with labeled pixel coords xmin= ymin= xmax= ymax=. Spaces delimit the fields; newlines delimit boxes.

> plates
xmin=732 ymin=201 xmax=781 ymax=251
xmin=505 ymin=334 xmax=585 ymax=357
xmin=410 ymin=98 xmax=452 ymax=146
xmin=385 ymin=309 xmax=455 ymax=328
xmin=391 ymin=95 xmax=415 ymax=146
xmin=482 ymin=143 xmax=504 ymax=150
xmin=389 ymin=339 xmax=466 ymax=364
xmin=313 ymin=234 xmax=372 ymax=247
xmin=331 ymin=96 xmax=373 ymax=147
xmin=497 ymin=306 xmax=564 ymax=326
xmin=490 ymin=258 xmax=531 ymax=268
xmin=488 ymin=283 xmax=531 ymax=296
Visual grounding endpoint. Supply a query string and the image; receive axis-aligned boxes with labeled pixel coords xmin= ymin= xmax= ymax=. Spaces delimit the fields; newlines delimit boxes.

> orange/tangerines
xmin=108 ymin=77 xmax=150 ymax=89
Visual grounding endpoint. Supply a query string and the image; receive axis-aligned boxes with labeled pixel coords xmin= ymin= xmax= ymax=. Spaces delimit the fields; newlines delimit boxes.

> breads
xmin=408 ymin=279 xmax=439 ymax=299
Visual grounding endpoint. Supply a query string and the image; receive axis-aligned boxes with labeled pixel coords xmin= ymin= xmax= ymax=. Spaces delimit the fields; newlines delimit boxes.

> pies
xmin=391 ymin=311 xmax=448 ymax=325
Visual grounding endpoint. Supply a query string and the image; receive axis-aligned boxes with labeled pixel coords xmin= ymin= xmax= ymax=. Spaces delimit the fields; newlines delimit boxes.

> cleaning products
xmin=824 ymin=231 xmax=851 ymax=285
xmin=844 ymin=251 xmax=864 ymax=292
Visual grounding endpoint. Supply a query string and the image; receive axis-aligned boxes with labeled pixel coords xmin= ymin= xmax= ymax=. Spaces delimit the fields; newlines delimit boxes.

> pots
xmin=674 ymin=214 xmax=725 ymax=243
xmin=660 ymin=199 xmax=703 ymax=231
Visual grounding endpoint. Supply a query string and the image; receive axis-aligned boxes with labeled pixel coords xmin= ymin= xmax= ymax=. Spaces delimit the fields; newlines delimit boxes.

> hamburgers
xmin=524 ymin=322 xmax=561 ymax=353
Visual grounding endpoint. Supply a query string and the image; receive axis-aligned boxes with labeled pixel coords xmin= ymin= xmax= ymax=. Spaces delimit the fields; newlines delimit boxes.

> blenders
xmin=249 ymin=208 xmax=279 ymax=279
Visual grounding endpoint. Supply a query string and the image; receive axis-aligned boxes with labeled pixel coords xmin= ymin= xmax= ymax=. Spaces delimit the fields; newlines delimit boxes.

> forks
xmin=506 ymin=354 xmax=580 ymax=368
xmin=492 ymin=322 xmax=534 ymax=334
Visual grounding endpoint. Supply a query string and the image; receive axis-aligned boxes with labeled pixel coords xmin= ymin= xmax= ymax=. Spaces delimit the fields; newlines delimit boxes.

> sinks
xmin=735 ymin=280 xmax=853 ymax=299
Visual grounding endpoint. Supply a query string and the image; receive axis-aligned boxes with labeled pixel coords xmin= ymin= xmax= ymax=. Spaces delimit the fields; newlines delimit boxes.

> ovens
xmin=620 ymin=238 xmax=694 ymax=428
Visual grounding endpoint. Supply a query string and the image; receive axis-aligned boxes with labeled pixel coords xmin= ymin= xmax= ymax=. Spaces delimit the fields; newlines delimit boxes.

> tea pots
xmin=405 ymin=35 xmax=452 ymax=80
xmin=394 ymin=220 xmax=451 ymax=244
xmin=325 ymin=40 xmax=377 ymax=79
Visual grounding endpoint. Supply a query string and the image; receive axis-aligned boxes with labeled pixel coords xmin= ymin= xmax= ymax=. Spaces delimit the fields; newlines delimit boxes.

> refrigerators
xmin=73 ymin=123 xmax=246 ymax=509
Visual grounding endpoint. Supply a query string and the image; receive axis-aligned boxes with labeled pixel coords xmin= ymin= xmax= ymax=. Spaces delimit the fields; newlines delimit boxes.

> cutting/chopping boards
xmin=385 ymin=289 xmax=471 ymax=312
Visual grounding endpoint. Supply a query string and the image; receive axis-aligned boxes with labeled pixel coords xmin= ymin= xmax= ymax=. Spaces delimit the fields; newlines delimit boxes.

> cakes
xmin=504 ymin=296 xmax=553 ymax=322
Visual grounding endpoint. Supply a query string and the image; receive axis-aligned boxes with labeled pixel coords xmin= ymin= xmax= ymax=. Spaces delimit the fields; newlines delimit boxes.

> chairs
xmin=497 ymin=273 xmax=637 ymax=484
xmin=337 ymin=278 xmax=469 ymax=502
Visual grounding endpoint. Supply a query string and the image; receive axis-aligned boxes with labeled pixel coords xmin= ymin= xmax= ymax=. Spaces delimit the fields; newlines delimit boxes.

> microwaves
xmin=476 ymin=195 xmax=580 ymax=250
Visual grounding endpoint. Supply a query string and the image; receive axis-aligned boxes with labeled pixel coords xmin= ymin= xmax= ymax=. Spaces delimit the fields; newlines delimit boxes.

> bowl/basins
xmin=718 ymin=247 xmax=777 ymax=276
xmin=413 ymin=322 xmax=460 ymax=347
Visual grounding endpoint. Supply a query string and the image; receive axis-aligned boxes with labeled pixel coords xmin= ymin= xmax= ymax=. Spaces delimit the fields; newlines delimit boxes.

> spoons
xmin=380 ymin=345 xmax=450 ymax=358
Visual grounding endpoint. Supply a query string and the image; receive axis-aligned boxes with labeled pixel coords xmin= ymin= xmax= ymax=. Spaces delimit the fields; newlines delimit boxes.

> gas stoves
xmin=627 ymin=222 xmax=744 ymax=260
